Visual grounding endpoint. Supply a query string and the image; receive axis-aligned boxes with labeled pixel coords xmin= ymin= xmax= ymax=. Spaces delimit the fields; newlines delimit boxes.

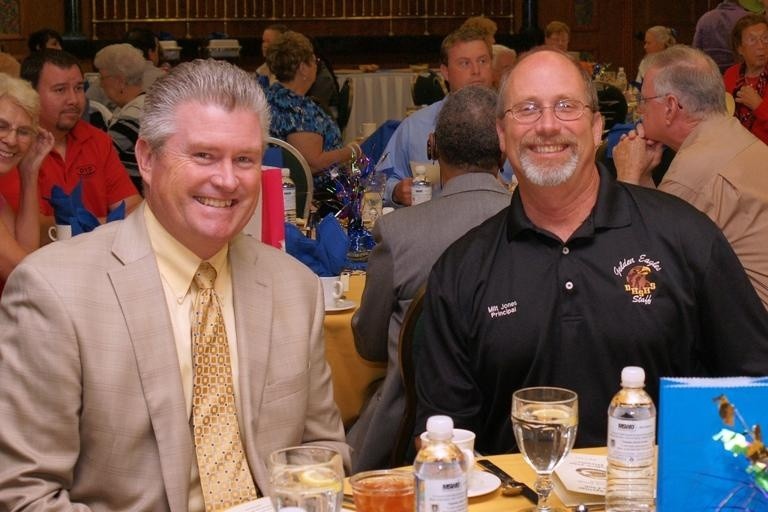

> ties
xmin=191 ymin=262 xmax=267 ymax=512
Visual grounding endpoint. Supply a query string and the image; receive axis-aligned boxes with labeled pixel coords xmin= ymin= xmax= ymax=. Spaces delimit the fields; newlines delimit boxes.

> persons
xmin=345 ymin=86 xmax=512 ymax=475
xmin=0 ymin=29 xmax=369 ymax=288
xmin=418 ymin=53 xmax=768 ymax=460
xmin=0 ymin=58 xmax=356 ymax=511
xmin=368 ymin=0 xmax=768 ymax=311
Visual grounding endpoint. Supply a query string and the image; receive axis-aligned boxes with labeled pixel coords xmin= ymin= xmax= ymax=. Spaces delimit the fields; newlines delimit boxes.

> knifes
xmin=477 ymin=458 xmax=542 ymax=504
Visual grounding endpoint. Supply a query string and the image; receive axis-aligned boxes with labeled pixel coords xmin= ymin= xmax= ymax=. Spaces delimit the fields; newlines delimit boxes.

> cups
xmin=48 ymin=225 xmax=72 ymax=240
xmin=416 ymin=429 xmax=477 ymax=462
xmin=350 ymin=469 xmax=418 ymax=511
xmin=314 ymin=275 xmax=344 ymax=306
xmin=359 ymin=123 xmax=377 ymax=136
xmin=265 ymin=446 xmax=343 ymax=511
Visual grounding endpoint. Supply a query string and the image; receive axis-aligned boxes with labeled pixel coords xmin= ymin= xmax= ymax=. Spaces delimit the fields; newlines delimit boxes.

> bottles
xmin=414 ymin=416 xmax=468 ymax=510
xmin=411 ymin=166 xmax=433 ymax=205
xmin=280 ymin=169 xmax=296 ymax=230
xmin=606 ymin=364 xmax=657 ymax=510
xmin=617 ymin=66 xmax=628 ymax=88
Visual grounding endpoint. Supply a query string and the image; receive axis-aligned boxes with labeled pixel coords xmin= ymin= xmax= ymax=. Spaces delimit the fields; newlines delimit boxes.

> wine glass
xmin=362 ymin=174 xmax=386 ymax=228
xmin=511 ymin=386 xmax=580 ymax=512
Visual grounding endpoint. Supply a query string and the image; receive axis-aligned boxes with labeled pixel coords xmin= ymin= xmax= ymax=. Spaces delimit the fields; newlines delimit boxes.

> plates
xmin=465 ymin=474 xmax=500 ymax=497
xmin=316 ymin=302 xmax=361 ymax=311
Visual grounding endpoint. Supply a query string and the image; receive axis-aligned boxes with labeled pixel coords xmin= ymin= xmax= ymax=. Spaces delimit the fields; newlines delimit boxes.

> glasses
xmin=636 ymin=93 xmax=686 ymax=109
xmin=503 ymin=99 xmax=593 ymax=122
